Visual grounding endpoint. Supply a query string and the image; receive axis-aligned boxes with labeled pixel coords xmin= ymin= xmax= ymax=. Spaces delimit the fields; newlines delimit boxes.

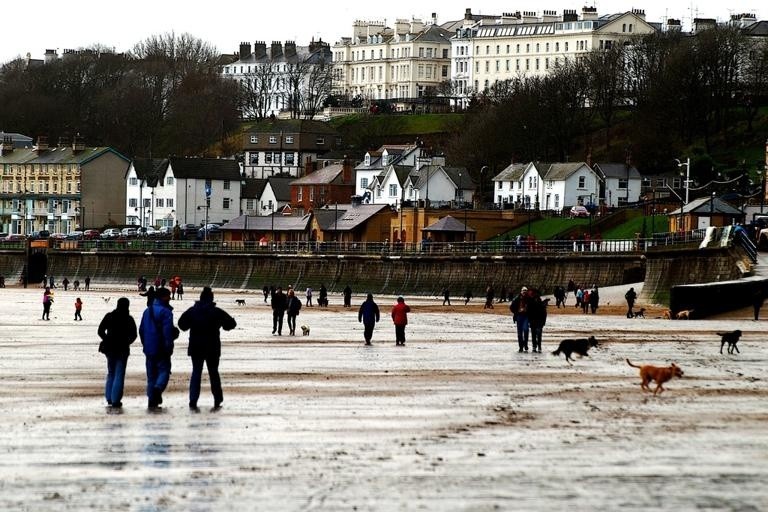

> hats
xmin=521 ymin=286 xmax=529 ymax=292
xmin=200 ymin=286 xmax=214 ymax=302
xmin=527 ymin=289 xmax=535 ymax=298
xmin=155 ymin=287 xmax=171 ymax=299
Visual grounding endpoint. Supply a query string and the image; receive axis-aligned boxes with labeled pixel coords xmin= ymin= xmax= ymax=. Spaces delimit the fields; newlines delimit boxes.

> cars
xmin=1 ymin=224 xmax=220 ymax=245
xmin=591 ymin=206 xmax=612 ymax=219
xmin=570 ymin=205 xmax=591 ymax=218
xmin=754 ymin=215 xmax=768 ymax=249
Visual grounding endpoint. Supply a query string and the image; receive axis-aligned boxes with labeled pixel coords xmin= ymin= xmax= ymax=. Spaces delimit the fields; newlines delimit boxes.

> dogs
xmin=551 ymin=335 xmax=602 ymax=366
xmin=101 ymin=296 xmax=111 ymax=303
xmin=634 ymin=308 xmax=646 ymax=319
xmin=675 ymin=310 xmax=694 ymax=319
xmin=626 ymin=358 xmax=685 ymax=396
xmin=300 ymin=325 xmax=311 ymax=335
xmin=715 ymin=329 xmax=743 ymax=355
xmin=235 ymin=298 xmax=246 ymax=306
xmin=662 ymin=309 xmax=671 ymax=319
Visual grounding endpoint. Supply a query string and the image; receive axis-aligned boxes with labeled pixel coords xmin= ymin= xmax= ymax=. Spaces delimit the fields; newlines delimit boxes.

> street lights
xmin=709 ymin=191 xmax=718 ymax=225
xmin=588 ymin=193 xmax=598 ymax=238
xmin=677 ymin=156 xmax=691 ymax=203
xmin=197 ymin=206 xmax=210 ymax=241
xmin=75 ymin=206 xmax=85 ymax=232
xmin=323 ymin=201 xmax=339 ymax=240
xmin=134 ymin=206 xmax=145 ymax=241
xmin=519 ymin=195 xmax=531 ymax=238
xmin=263 ymin=203 xmax=275 ymax=242
xmin=457 ymin=172 xmax=463 ymax=203
xmin=755 ymin=168 xmax=767 ymax=213
xmin=455 ymin=200 xmax=467 ymax=252
xmin=389 ymin=201 xmax=404 ymax=242
xmin=678 ymin=172 xmax=684 ymax=231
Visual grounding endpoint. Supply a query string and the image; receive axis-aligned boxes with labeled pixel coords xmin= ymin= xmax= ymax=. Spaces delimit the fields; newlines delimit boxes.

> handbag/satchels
xmin=98 ymin=341 xmax=105 ymax=353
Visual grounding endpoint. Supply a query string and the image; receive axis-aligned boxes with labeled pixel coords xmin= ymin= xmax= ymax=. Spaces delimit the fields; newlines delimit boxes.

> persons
xmin=392 ymin=296 xmax=410 ymax=346
xmin=138 ymin=275 xmax=185 ymax=300
xmin=41 ymin=286 xmax=54 ymax=321
xmin=753 ymin=286 xmax=763 ymax=321
xmin=285 ymin=288 xmax=302 ymax=336
xmin=0 ymin=270 xmax=91 ymax=291
xmin=269 ymin=286 xmax=287 ymax=335
xmin=95 ymin=295 xmax=137 ymax=405
xmin=358 ymin=293 xmax=380 ymax=346
xmin=442 ymin=278 xmax=638 ymax=319
xmin=179 ymin=287 xmax=237 ymax=412
xmin=263 ymin=285 xmax=353 ymax=309
xmin=509 ymin=284 xmax=532 ymax=354
xmin=74 ymin=297 xmax=83 ymax=321
xmin=525 ymin=290 xmax=547 ymax=355
xmin=140 ymin=286 xmax=178 ymax=407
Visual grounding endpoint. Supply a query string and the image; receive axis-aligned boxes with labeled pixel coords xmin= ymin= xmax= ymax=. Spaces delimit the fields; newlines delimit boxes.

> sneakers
xmin=189 ymin=402 xmax=201 ymax=413
xmin=148 ymin=406 xmax=162 ymax=413
xmin=517 ymin=349 xmax=542 ymax=353
xmin=152 ymin=386 xmax=163 ymax=405
xmin=272 ymin=330 xmax=296 ymax=336
xmin=107 ymin=399 xmax=123 ymax=408
